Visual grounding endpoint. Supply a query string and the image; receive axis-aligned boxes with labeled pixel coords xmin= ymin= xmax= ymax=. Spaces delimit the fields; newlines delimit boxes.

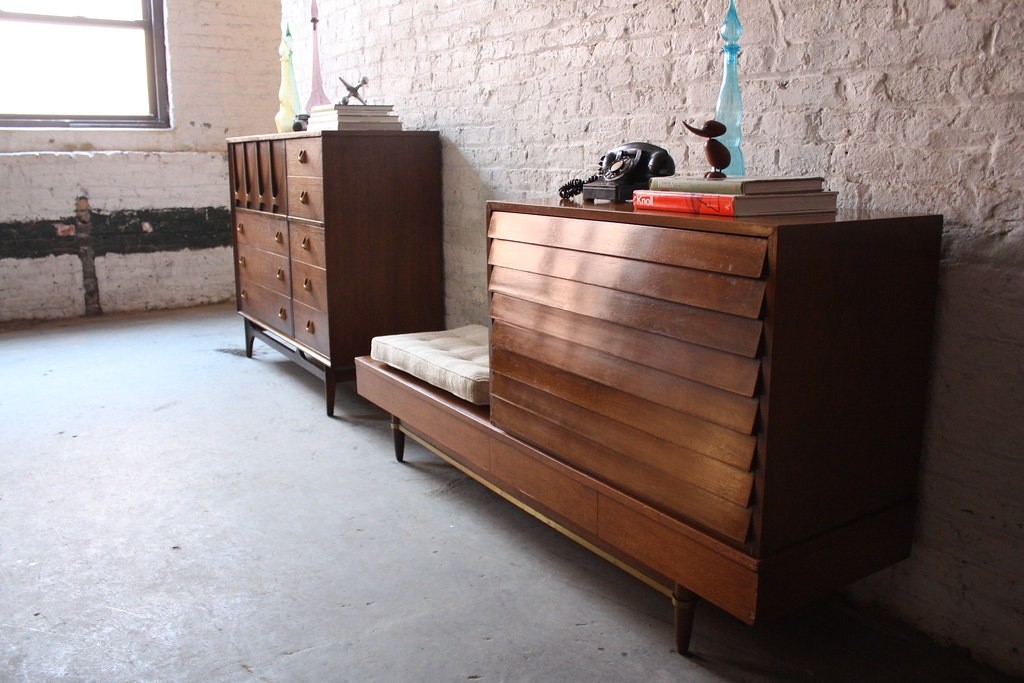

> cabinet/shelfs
xmin=224 ymin=129 xmax=444 ymax=417
xmin=352 ymin=199 xmax=943 ymax=654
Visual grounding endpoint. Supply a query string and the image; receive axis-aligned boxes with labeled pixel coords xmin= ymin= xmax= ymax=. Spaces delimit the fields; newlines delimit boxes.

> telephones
xmin=582 ymin=141 xmax=675 ymax=204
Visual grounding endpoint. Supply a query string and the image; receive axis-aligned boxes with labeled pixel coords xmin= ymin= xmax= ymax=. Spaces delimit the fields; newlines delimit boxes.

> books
xmin=307 ymin=104 xmax=404 ymax=131
xmin=633 ymin=176 xmax=839 ymax=217
